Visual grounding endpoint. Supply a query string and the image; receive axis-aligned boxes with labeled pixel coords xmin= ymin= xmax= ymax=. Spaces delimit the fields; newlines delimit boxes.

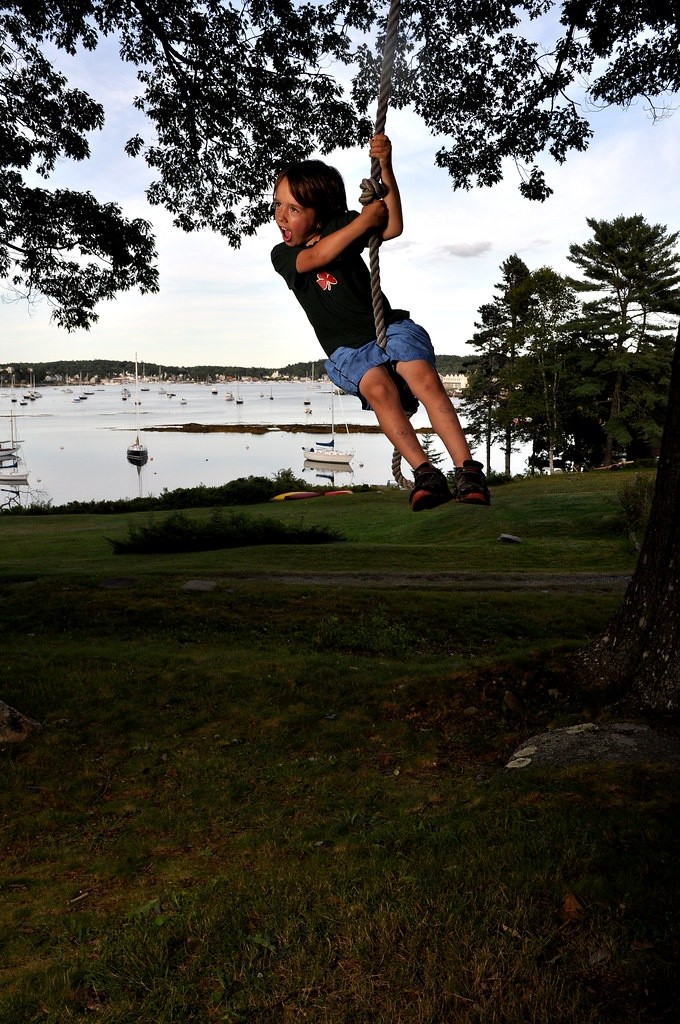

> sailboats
xmin=0 ymin=480 xmax=30 ymax=505
xmin=124 ymin=349 xmax=149 ymax=458
xmin=10 ymin=369 xmax=42 ymax=405
xmin=301 ymin=459 xmax=355 ymax=487
xmin=300 ymin=381 xmax=358 ymax=464
xmin=127 ymin=457 xmax=148 ymax=498
xmin=0 ymin=409 xmax=25 ymax=456
xmin=62 ymin=361 xmax=315 ymax=415
xmin=0 ymin=416 xmax=29 ymax=481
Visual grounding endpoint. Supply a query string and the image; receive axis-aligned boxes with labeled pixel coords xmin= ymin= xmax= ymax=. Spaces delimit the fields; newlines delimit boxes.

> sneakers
xmin=409 ymin=462 xmax=454 ymax=511
xmin=453 ymin=459 xmax=491 ymax=505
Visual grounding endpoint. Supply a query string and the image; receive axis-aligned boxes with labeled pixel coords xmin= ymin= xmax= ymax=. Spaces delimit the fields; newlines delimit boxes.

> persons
xmin=271 ymin=134 xmax=490 ymax=511
